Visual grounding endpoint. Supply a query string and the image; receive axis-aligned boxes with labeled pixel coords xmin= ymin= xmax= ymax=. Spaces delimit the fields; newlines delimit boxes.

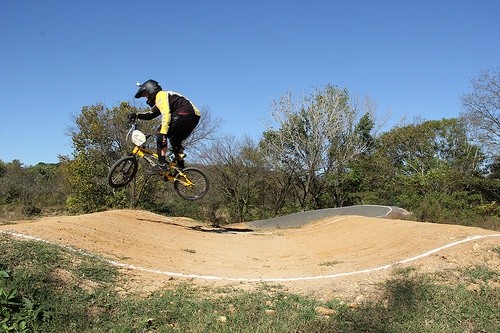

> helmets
xmin=135 ymin=80 xmax=162 ymax=107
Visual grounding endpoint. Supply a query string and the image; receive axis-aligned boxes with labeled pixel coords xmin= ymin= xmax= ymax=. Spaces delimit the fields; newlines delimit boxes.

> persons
xmin=134 ymin=79 xmax=201 ymax=173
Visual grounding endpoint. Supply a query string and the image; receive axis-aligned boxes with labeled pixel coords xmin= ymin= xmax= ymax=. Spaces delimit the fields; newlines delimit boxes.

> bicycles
xmin=108 ymin=113 xmax=210 ymax=201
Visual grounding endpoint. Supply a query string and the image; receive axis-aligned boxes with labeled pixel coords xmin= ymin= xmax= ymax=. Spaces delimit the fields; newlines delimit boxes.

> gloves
xmin=128 ymin=113 xmax=137 ymax=121
xmin=158 ymin=134 xmax=166 ymax=142
